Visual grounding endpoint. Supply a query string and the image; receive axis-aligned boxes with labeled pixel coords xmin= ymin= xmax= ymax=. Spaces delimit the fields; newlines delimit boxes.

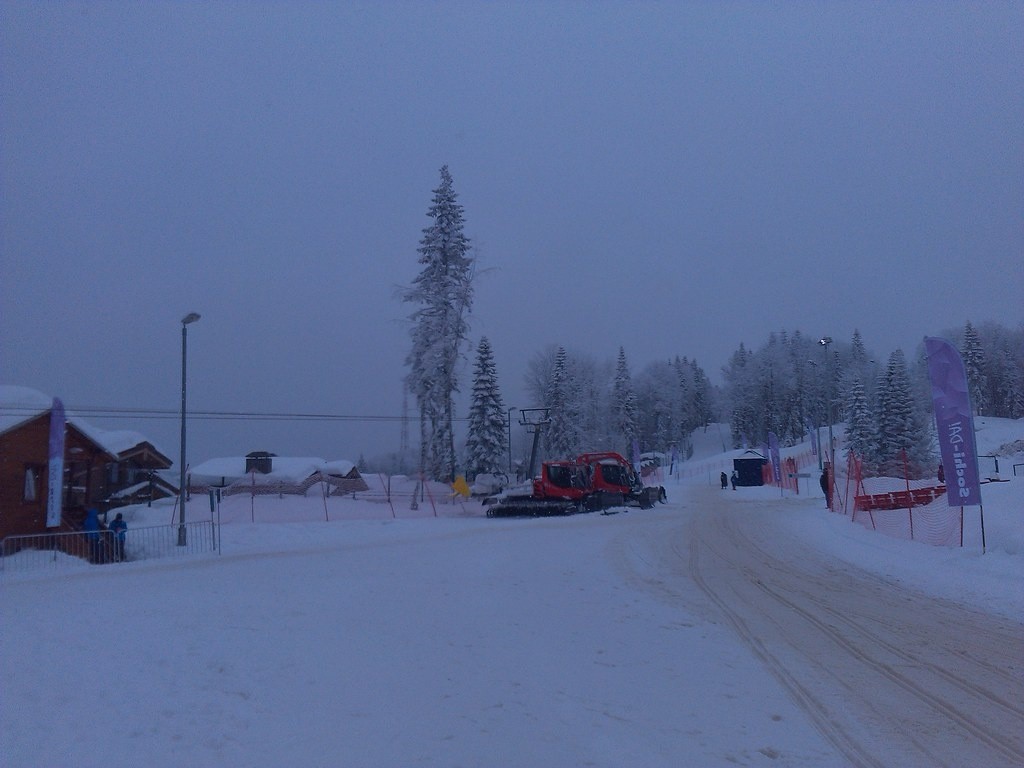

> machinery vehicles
xmin=484 ymin=450 xmax=667 ymax=518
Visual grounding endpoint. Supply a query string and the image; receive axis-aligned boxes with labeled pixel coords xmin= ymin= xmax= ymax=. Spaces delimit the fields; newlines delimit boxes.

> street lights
xmin=507 ymin=406 xmax=517 ymax=472
xmin=817 ymin=336 xmax=833 ymax=460
xmin=176 ymin=312 xmax=203 ymax=545
xmin=807 ymin=359 xmax=822 ymax=469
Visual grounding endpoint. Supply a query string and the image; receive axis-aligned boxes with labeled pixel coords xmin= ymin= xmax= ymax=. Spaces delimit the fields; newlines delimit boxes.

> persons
xmin=721 ymin=472 xmax=727 ymax=489
xmin=938 ymin=465 xmax=945 ymax=483
xmin=820 ymin=468 xmax=829 ymax=508
xmin=109 ymin=513 xmax=127 ymax=563
xmin=731 ymin=474 xmax=738 ymax=490
xmin=84 ymin=508 xmax=100 ymax=564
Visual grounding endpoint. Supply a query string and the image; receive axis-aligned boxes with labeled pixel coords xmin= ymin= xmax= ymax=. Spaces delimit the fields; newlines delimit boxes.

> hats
xmin=822 ymin=467 xmax=828 ymax=473
xmin=117 ymin=513 xmax=122 ymax=519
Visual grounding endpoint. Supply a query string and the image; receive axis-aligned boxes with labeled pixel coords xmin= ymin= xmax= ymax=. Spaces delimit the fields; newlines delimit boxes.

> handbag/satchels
xmin=720 ymin=479 xmax=727 ymax=484
